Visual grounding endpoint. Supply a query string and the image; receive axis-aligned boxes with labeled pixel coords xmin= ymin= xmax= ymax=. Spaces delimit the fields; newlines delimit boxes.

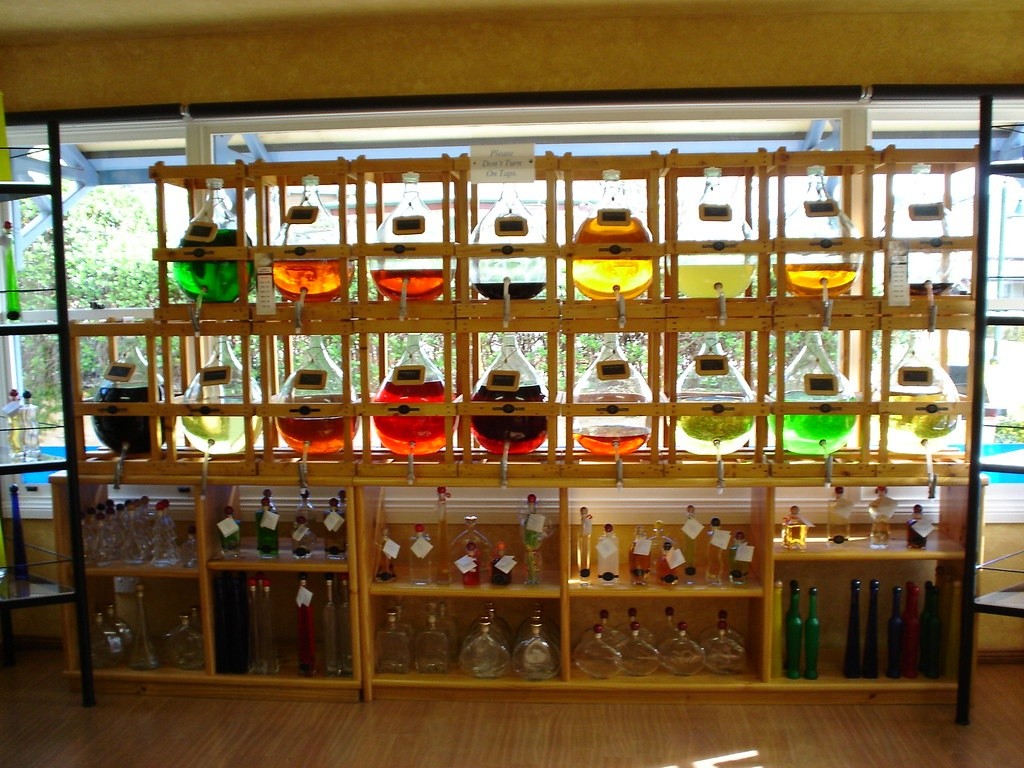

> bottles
xmin=470 ymin=333 xmax=548 ymax=455
xmin=272 ymin=176 xmax=354 ymax=302
xmin=767 ymin=330 xmax=857 ymax=455
xmin=560 ymin=333 xmax=652 ymax=456
xmin=173 ymin=178 xmax=256 ymax=303
xmin=571 ymin=167 xmax=654 ymax=300
xmin=272 ymin=335 xmax=360 ymax=454
xmin=84 ymin=483 xmax=944 ymax=680
xmin=666 ymin=167 xmax=757 ymax=298
xmin=879 ymin=164 xmax=969 ymax=296
xmin=90 ymin=317 xmax=176 ymax=454
xmin=469 ymin=182 xmax=547 ymax=300
xmin=180 ymin=336 xmax=263 ymax=455
xmin=772 ymin=164 xmax=861 ymax=297
xmin=871 ymin=330 xmax=961 ymax=455
xmin=371 ymin=333 xmax=458 ymax=456
xmin=367 ymin=172 xmax=456 ymax=301
xmin=667 ymin=331 xmax=755 ymax=455
xmin=7 ymin=389 xmax=44 ymax=460
xmin=1 ymin=225 xmax=22 ymax=321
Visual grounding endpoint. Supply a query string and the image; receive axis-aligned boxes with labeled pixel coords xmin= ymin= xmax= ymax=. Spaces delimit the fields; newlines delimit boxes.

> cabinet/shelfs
xmin=0 ymin=144 xmax=1024 ymax=725
